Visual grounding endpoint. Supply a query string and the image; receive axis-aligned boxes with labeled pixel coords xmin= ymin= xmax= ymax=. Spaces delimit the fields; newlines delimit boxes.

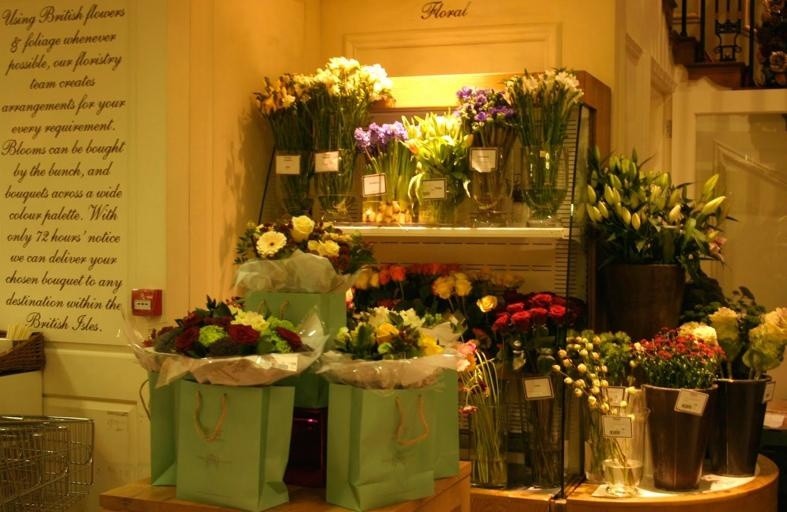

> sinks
xmin=1 ymin=330 xmax=12 ymax=357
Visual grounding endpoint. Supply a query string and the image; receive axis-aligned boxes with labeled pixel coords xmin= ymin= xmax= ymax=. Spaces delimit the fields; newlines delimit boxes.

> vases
xmin=601 ymin=261 xmax=687 ymax=345
xmin=703 ymin=372 xmax=773 ymax=478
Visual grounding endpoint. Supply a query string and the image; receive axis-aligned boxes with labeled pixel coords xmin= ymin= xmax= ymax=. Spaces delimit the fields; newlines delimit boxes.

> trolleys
xmin=0 ymin=412 xmax=97 ymax=510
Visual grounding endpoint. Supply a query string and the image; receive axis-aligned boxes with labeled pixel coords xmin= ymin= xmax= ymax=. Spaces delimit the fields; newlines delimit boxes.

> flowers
xmin=574 ymin=144 xmax=731 ymax=289
xmin=678 ymin=284 xmax=785 ymax=380
xmin=538 ymin=326 xmax=649 ymax=493
xmin=231 ymin=209 xmax=379 ymax=295
xmin=251 ymin=59 xmax=587 ymax=214
xmin=335 ymin=260 xmax=583 ymax=363
xmin=629 ymin=328 xmax=729 ymax=393
xmin=137 ymin=292 xmax=317 ymax=362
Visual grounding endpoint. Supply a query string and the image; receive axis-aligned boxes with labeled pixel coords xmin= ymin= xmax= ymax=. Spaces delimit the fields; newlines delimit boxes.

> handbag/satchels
xmin=150 ymin=373 xmax=196 ymax=486
xmin=325 ymin=383 xmax=435 ymax=510
xmin=245 ymin=292 xmax=347 ymax=408
xmin=434 ymin=368 xmax=460 ymax=480
xmin=173 ymin=378 xmax=296 ymax=511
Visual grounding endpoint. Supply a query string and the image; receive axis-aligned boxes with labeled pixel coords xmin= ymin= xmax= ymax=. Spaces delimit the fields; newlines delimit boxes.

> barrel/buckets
xmin=613 ymin=260 xmax=687 ymax=342
xmin=710 ymin=378 xmax=771 ymax=476
